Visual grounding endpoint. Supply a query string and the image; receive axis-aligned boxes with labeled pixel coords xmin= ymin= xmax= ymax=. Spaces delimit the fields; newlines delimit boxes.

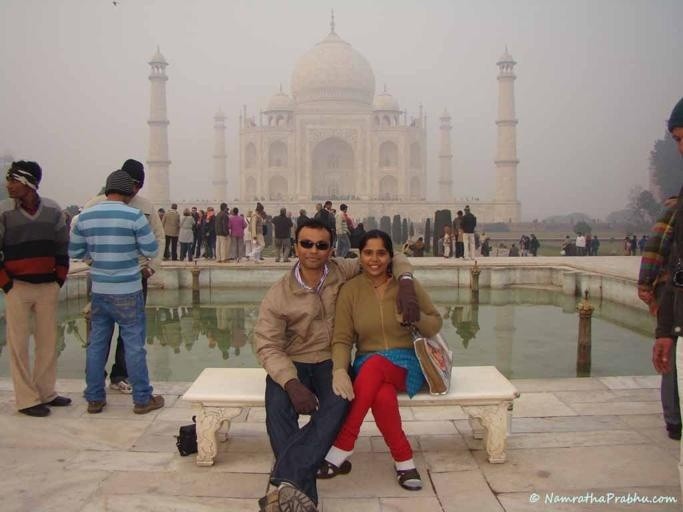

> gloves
xmin=284 ymin=378 xmax=318 ymax=415
xmin=396 ymin=279 xmax=419 ymax=323
xmin=333 ymin=369 xmax=354 ymax=401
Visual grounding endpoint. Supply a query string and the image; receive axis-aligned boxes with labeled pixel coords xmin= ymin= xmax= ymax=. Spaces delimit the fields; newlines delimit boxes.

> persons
xmin=631 ymin=236 xmax=637 ymax=256
xmin=179 ymin=208 xmax=195 ymax=261
xmin=158 ymin=208 xmax=165 ymax=219
xmin=530 ymin=234 xmax=539 ymax=256
xmin=625 ymin=237 xmax=631 ymax=256
xmin=453 ymin=211 xmax=464 ymax=258
xmin=191 ymin=207 xmax=197 ymax=221
xmin=460 ymin=205 xmax=476 ymax=260
xmin=413 ymin=237 xmax=426 ymax=257
xmin=205 ymin=207 xmax=215 ymax=258
xmin=562 ymin=236 xmax=572 ymax=255
xmin=316 ymin=203 xmax=322 ymax=211
xmin=481 ymin=238 xmax=492 ymax=257
xmin=585 ymin=235 xmax=591 ymax=256
xmin=315 ymin=201 xmax=336 ymax=228
xmin=639 ymin=236 xmax=647 ymax=253
xmin=317 ymin=230 xmax=443 ymax=490
xmin=272 ymin=208 xmax=293 ymax=262
xmin=652 ymin=99 xmax=683 ymax=432
xmin=82 ymin=159 xmax=165 ymax=396
xmin=255 ymin=219 xmax=419 ymax=511
xmin=576 ymin=232 xmax=585 ymax=256
xmin=351 ymin=223 xmax=367 ymax=248
xmin=592 ymin=236 xmax=600 ymax=257
xmin=297 ymin=209 xmax=311 ymax=224
xmin=216 ymin=203 xmax=229 ymax=262
xmin=67 ymin=171 xmax=165 ymax=414
xmin=521 ymin=236 xmax=529 ymax=256
xmin=1 ymin=161 xmax=71 ymax=415
xmin=639 ymin=192 xmax=681 ymax=440
xmin=244 ymin=210 xmax=253 ymax=257
xmin=229 ymin=208 xmax=247 ymax=261
xmin=248 ymin=203 xmax=266 ymax=264
xmin=335 ymin=204 xmax=351 ymax=258
xmin=509 ymin=244 xmax=519 ymax=256
xmin=192 ymin=210 xmax=205 ymax=258
xmin=162 ymin=204 xmax=180 ymax=261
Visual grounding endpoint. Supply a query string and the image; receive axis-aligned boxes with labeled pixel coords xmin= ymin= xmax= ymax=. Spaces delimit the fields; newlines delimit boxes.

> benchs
xmin=182 ymin=366 xmax=520 ymax=466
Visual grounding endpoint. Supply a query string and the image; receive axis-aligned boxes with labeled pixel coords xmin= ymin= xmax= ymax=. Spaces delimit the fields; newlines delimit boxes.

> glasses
xmin=298 ymin=239 xmax=331 ymax=250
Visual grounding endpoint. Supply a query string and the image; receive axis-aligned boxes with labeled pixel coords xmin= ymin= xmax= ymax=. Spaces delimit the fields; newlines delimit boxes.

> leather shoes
xmin=666 ymin=424 xmax=682 ymax=440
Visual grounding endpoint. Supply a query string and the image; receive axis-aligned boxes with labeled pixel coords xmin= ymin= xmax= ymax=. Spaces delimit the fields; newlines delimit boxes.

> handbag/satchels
xmin=176 ymin=415 xmax=199 ymax=457
xmin=407 ymin=323 xmax=452 ymax=396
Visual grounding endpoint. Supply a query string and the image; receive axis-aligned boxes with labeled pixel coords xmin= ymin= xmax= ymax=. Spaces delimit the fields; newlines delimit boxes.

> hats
xmin=105 ymin=171 xmax=133 ymax=193
xmin=464 ymin=205 xmax=470 ymax=211
xmin=668 ymin=99 xmax=683 ymax=131
xmin=255 ymin=202 xmax=264 ymax=209
xmin=122 ymin=159 xmax=144 ymax=185
xmin=6 ymin=161 xmax=42 ymax=190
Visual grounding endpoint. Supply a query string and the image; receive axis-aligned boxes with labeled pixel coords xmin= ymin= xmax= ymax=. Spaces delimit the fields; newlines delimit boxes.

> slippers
xmin=392 ymin=463 xmax=422 ymax=490
xmin=316 ymin=459 xmax=352 ymax=479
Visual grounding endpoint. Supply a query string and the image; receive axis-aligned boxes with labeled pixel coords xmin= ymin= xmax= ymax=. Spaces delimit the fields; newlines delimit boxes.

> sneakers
xmin=87 ymin=400 xmax=106 ymax=413
xmin=259 ymin=489 xmax=281 ymax=512
xmin=134 ymin=396 xmax=165 ymax=414
xmin=18 ymin=405 xmax=49 ymax=417
xmin=44 ymin=397 xmax=71 ymax=406
xmin=278 ymin=486 xmax=319 ymax=512
xmin=111 ymin=379 xmax=133 ymax=394
